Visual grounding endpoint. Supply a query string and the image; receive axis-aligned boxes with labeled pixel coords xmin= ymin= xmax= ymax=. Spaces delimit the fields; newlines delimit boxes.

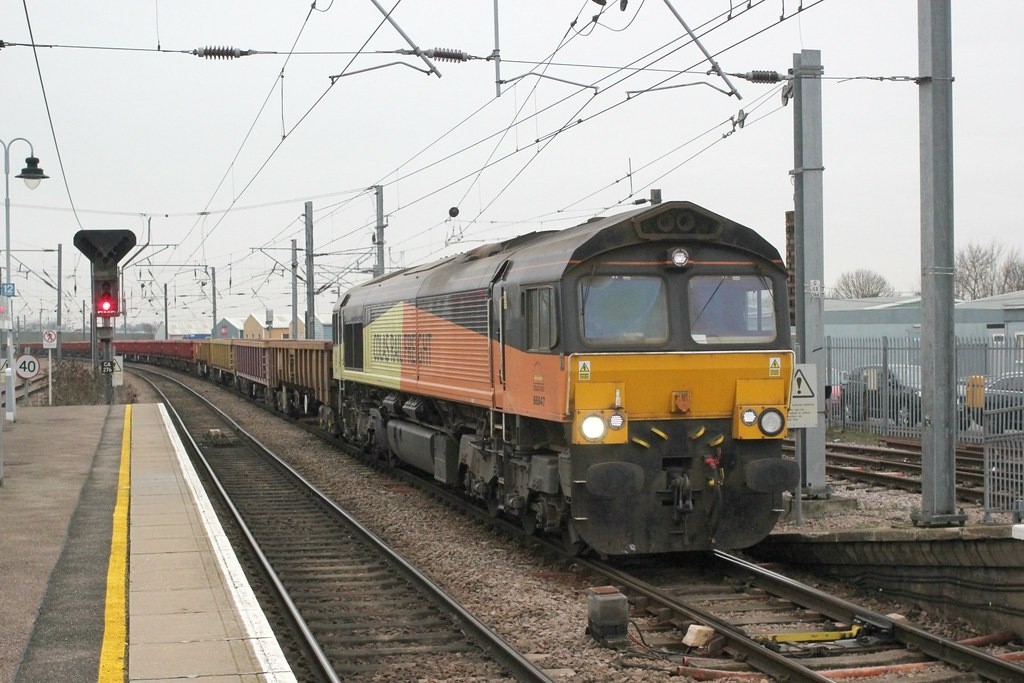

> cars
xmin=826 ymin=366 xmax=846 ymax=420
xmin=845 ymin=364 xmax=973 ymax=429
xmin=971 ymin=370 xmax=1024 ymax=435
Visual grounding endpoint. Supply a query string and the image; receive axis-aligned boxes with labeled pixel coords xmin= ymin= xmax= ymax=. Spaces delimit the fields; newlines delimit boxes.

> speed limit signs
xmin=16 ymin=354 xmax=40 ymax=379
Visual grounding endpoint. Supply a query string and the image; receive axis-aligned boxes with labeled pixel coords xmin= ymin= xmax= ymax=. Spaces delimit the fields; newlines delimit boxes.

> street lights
xmin=1 ymin=137 xmax=51 ymax=422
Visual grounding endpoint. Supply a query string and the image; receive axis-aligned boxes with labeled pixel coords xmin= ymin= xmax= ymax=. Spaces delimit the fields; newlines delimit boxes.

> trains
xmin=3 ymin=188 xmax=798 ymax=562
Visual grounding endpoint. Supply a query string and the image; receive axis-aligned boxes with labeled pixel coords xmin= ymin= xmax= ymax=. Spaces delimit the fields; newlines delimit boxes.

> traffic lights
xmin=95 ymin=280 xmax=118 ymax=318
